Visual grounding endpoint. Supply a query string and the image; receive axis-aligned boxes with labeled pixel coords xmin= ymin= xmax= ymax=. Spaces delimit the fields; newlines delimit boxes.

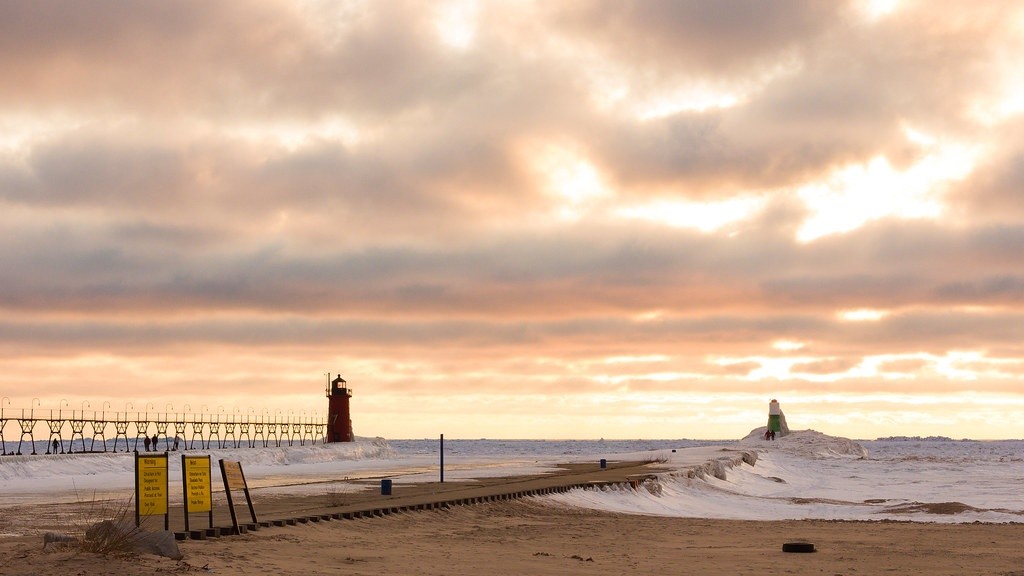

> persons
xmin=770 ymin=430 xmax=775 ymax=440
xmin=176 ymin=435 xmax=179 ymax=449
xmin=52 ymin=438 xmax=59 ymax=454
xmin=144 ymin=436 xmax=151 ymax=451
xmin=765 ymin=430 xmax=770 ymax=440
xmin=152 ymin=434 xmax=158 ymax=450
